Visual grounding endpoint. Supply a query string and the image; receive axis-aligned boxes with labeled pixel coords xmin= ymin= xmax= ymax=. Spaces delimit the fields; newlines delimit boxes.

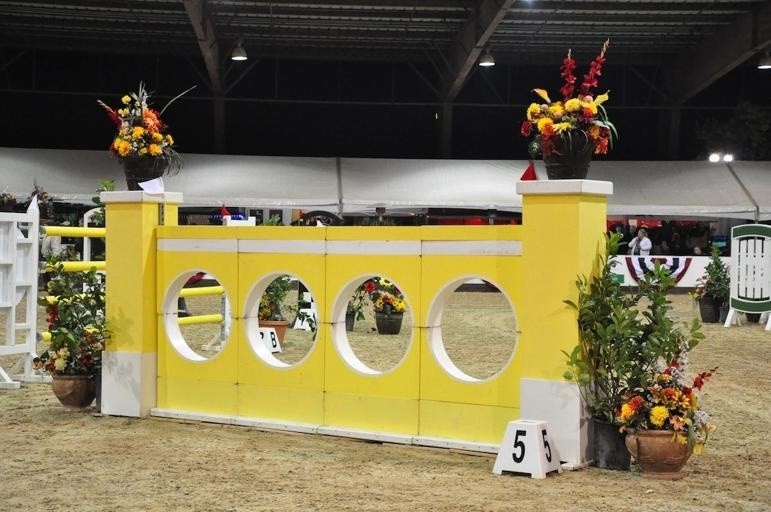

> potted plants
xmin=688 ymin=242 xmax=732 ymax=324
xmin=346 ymin=286 xmax=370 ymax=332
xmin=257 ymin=272 xmax=318 ymax=354
xmin=557 ymin=225 xmax=709 ymax=474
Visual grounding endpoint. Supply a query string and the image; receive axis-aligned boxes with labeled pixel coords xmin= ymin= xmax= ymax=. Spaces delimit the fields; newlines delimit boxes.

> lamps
xmin=231 ymin=36 xmax=249 ymax=63
xmin=476 ymin=43 xmax=497 ymax=68
xmin=750 ymin=43 xmax=771 ymax=72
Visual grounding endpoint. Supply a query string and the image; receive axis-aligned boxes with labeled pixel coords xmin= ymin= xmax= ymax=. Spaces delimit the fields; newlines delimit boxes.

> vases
xmin=621 ymin=427 xmax=697 ymax=477
xmin=50 ymin=376 xmax=97 ymax=410
xmin=120 ymin=154 xmax=168 ymax=190
xmin=374 ymin=308 xmax=406 ymax=334
xmin=546 ymin=135 xmax=595 ymax=179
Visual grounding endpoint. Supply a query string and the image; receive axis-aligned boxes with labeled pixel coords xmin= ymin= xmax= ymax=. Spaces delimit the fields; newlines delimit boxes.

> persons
xmin=628 ymin=229 xmax=653 ymax=255
xmin=38 ymin=219 xmax=62 ymax=292
xmin=653 ymin=232 xmax=707 ymax=255
xmin=74 ymin=218 xmax=84 ymax=260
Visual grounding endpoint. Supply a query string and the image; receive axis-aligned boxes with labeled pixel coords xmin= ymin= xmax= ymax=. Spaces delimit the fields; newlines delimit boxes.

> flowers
xmin=519 ymin=33 xmax=624 ymax=161
xmin=95 ymin=79 xmax=200 ymax=175
xmin=613 ymin=336 xmax=723 ymax=459
xmin=363 ymin=273 xmax=409 ymax=322
xmin=30 ymin=241 xmax=117 ymax=376
